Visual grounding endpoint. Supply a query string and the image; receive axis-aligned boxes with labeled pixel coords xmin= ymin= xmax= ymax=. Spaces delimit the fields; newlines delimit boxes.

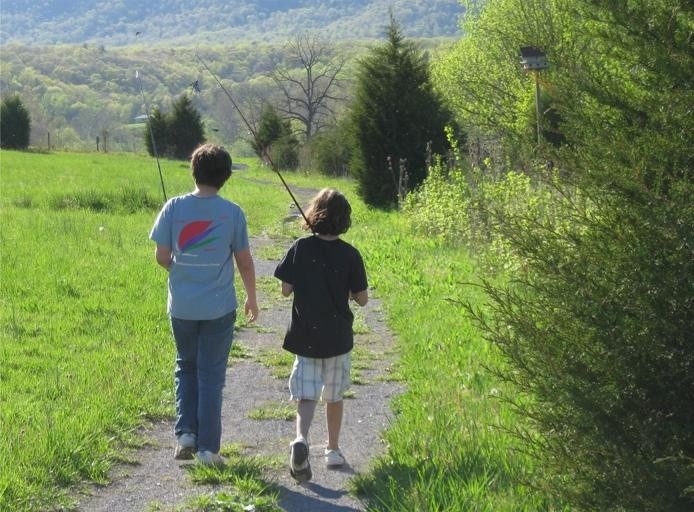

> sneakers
xmin=289 ymin=438 xmax=313 ymax=481
xmin=323 ymin=447 xmax=346 ymax=466
xmin=194 ymin=450 xmax=230 ymax=467
xmin=173 ymin=431 xmax=196 ymax=461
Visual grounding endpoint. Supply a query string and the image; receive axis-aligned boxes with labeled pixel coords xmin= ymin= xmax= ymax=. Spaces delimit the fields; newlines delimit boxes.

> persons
xmin=270 ymin=186 xmax=367 ymax=481
xmin=147 ymin=142 xmax=259 ymax=469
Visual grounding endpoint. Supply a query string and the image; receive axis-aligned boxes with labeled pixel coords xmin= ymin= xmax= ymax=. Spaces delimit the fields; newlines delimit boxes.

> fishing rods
xmin=196 ymin=52 xmax=318 ymax=236
xmin=136 ymin=69 xmax=167 ymax=204
